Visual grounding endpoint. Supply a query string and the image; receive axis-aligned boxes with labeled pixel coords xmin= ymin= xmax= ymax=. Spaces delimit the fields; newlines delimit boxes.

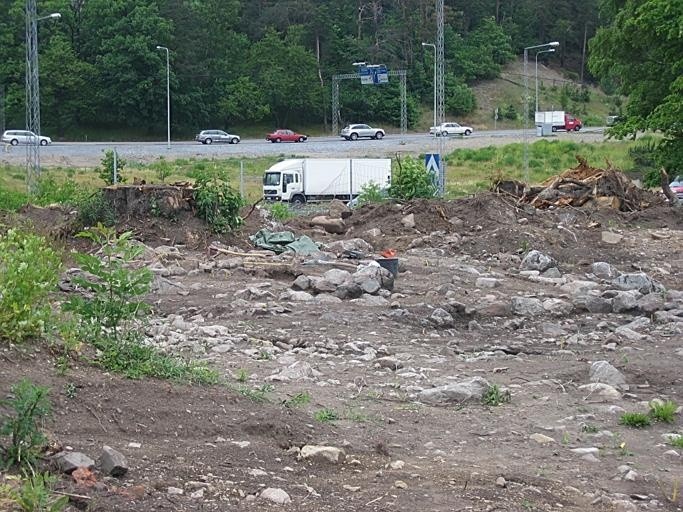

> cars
xmin=428 ymin=121 xmax=472 ymax=136
xmin=669 ymin=174 xmax=682 ymax=201
xmin=266 ymin=129 xmax=307 ymax=143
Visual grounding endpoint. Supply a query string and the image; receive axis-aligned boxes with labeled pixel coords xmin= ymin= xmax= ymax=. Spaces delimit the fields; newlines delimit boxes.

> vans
xmin=2 ymin=129 xmax=51 ymax=148
xmin=606 ymin=115 xmax=618 ymax=127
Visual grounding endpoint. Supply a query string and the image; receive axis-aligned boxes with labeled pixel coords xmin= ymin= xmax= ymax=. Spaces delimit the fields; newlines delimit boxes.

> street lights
xmin=421 ymin=42 xmax=436 ymax=139
xmin=534 ymin=48 xmax=555 ymax=113
xmin=156 ymin=46 xmax=171 ymax=149
xmin=33 ymin=11 xmax=61 ymax=22
xmin=520 ymin=41 xmax=559 ymax=186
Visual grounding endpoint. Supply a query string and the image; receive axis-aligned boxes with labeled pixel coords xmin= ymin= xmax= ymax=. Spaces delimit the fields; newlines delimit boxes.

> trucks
xmin=260 ymin=157 xmax=391 ymax=206
xmin=532 ymin=111 xmax=582 ymax=132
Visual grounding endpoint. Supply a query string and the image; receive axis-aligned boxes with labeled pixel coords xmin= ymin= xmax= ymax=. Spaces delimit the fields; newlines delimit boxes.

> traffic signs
xmin=358 ymin=65 xmax=388 ymax=85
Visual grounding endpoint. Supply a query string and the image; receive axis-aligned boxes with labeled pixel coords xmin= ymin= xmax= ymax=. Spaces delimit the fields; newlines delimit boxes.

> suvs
xmin=194 ymin=130 xmax=240 ymax=145
xmin=341 ymin=123 xmax=385 ymax=142
